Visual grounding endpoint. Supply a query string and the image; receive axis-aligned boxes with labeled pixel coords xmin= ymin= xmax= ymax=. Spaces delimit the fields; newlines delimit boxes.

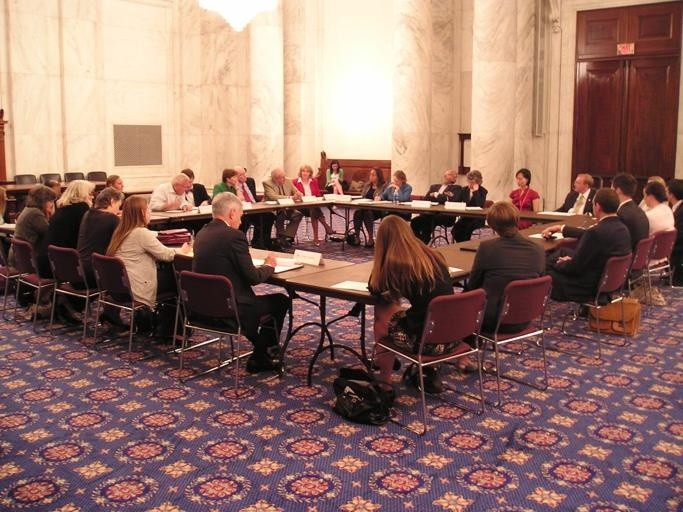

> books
xmin=251 ymin=256 xmax=304 ymax=274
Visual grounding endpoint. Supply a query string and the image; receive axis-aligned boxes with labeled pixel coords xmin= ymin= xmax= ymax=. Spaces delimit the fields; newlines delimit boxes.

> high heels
xmin=366 ymin=241 xmax=375 ymax=248
xmin=326 ymin=230 xmax=336 ymax=236
xmin=314 ymin=241 xmax=320 ymax=246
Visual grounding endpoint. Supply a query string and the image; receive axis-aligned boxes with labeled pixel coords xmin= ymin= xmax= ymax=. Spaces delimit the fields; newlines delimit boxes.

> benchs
xmin=316 ymin=149 xmax=392 ymax=197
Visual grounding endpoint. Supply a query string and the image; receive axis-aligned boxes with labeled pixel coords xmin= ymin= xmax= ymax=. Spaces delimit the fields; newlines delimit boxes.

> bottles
xmin=393 ymin=189 xmax=399 ymax=205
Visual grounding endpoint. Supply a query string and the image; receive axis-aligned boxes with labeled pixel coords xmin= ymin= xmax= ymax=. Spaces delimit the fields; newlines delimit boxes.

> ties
xmin=279 ymin=185 xmax=284 ymax=195
xmin=242 ymin=182 xmax=253 ymax=203
xmin=573 ymin=193 xmax=584 ymax=214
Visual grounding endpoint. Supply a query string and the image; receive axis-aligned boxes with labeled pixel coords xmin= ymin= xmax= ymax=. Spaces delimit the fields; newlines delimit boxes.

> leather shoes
xmin=248 ymin=359 xmax=282 ymax=372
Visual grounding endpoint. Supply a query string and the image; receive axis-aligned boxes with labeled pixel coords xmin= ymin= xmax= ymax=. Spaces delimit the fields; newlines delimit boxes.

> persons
xmin=369 ymin=215 xmax=462 ymax=399
xmin=540 ymin=172 xmax=683 ymax=317
xmin=456 ymin=201 xmax=547 ymax=372
xmin=149 ymin=168 xmax=212 ymax=235
xmin=507 ymin=168 xmax=541 ymax=230
xmin=0 ymin=175 xmax=193 ymax=345
xmin=352 ymin=167 xmax=412 ymax=248
xmin=409 ymin=169 xmax=489 ymax=245
xmin=194 ymin=191 xmax=289 ymax=373
xmin=325 ymin=160 xmax=345 ymax=196
xmin=212 ymin=165 xmax=336 ymax=251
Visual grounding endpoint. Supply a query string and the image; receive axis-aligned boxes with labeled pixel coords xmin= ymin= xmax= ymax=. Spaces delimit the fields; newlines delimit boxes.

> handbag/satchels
xmin=333 ymin=369 xmax=396 ymax=426
xmin=589 ymin=295 xmax=642 ymax=337
